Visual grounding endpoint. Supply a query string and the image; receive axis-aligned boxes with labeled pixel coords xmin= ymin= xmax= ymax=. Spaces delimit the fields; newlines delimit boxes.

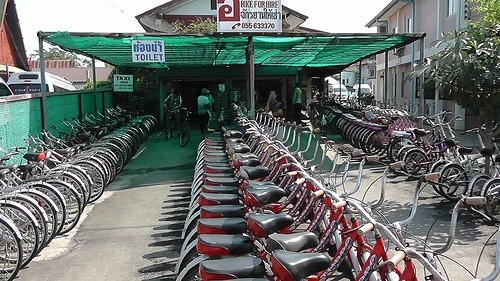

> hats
xmin=202 ymin=87 xmax=208 ymax=92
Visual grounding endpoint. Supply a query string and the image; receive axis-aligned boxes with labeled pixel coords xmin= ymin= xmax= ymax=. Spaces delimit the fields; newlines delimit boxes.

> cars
xmin=350 ymin=83 xmax=373 ymax=97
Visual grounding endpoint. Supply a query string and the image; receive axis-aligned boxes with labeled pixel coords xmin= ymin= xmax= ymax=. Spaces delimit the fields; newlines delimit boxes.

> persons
xmin=264 ymin=87 xmax=284 ymax=117
xmin=195 ymin=88 xmax=216 ymax=134
xmin=163 ymin=87 xmax=182 ymax=140
xmin=291 ymin=82 xmax=302 ymax=120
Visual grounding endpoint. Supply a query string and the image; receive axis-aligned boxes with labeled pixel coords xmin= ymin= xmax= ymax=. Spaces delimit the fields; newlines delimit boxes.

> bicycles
xmin=0 ymin=105 xmax=159 ymax=281
xmin=173 ymin=97 xmax=500 ymax=281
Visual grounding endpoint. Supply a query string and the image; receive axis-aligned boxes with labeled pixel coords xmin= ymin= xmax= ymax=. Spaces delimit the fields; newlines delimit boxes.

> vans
xmin=328 ymin=82 xmax=348 ymax=102
xmin=6 ymin=71 xmax=77 ymax=94
